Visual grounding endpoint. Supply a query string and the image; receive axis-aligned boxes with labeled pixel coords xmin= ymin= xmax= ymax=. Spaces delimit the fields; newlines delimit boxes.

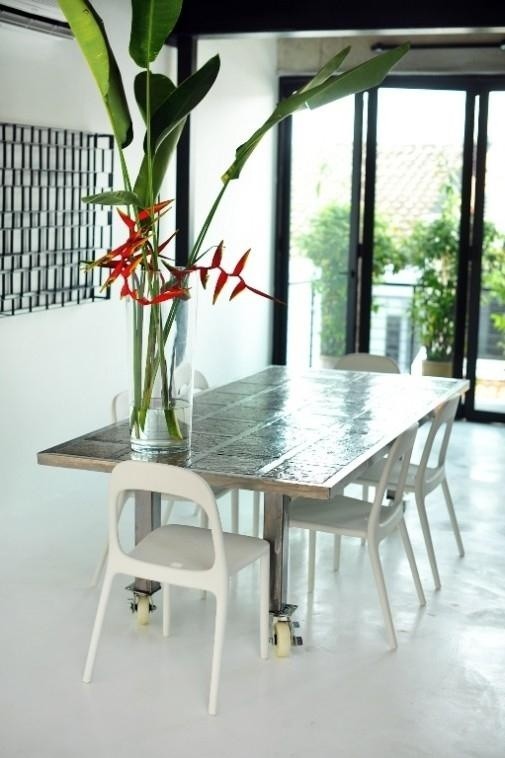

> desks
xmin=35 ymin=363 xmax=478 ymax=658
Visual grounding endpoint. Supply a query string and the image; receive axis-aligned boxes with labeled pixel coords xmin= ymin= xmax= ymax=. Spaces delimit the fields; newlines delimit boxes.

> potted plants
xmin=402 ymin=182 xmax=504 ymax=379
xmin=61 ymin=1 xmax=419 ymax=444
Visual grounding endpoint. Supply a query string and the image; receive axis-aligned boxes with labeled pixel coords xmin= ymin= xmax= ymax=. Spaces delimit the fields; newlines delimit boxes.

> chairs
xmin=334 ymin=395 xmax=464 ymax=590
xmin=93 ymin=387 xmax=232 ymax=598
xmin=84 ymin=461 xmax=269 ymax=714
xmin=287 ymin=421 xmax=426 ymax=650
xmin=160 ymin=357 xmax=261 ymax=539
xmin=333 ymin=353 xmax=401 ymax=373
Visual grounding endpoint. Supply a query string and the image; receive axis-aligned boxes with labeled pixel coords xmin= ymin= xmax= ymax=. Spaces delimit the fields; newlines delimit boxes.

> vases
xmin=123 ymin=265 xmax=197 ymax=454
